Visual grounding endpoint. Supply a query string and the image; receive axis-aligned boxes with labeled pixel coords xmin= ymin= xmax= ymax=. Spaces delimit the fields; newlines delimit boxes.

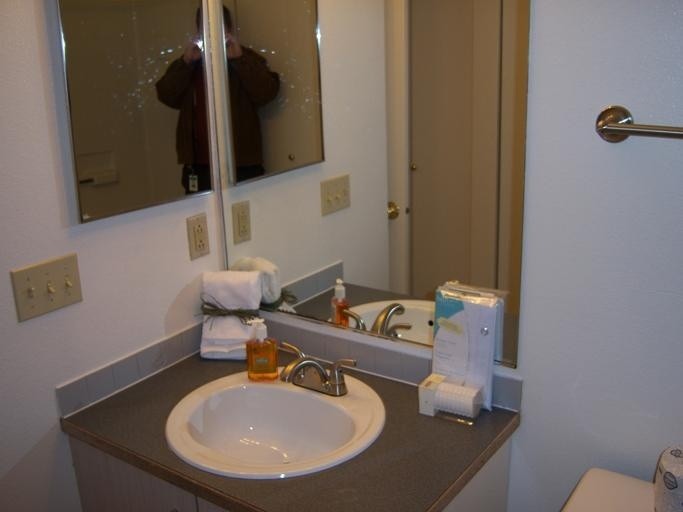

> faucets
xmin=280 ymin=357 xmax=329 ymax=383
xmin=371 ymin=303 xmax=404 ymax=336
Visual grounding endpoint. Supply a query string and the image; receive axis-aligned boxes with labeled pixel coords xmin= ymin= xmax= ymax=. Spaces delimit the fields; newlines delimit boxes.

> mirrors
xmin=204 ymin=0 xmax=532 ymax=416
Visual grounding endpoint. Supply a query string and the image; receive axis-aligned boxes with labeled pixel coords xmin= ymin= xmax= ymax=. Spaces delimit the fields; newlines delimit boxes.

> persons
xmin=154 ymin=4 xmax=280 ymax=198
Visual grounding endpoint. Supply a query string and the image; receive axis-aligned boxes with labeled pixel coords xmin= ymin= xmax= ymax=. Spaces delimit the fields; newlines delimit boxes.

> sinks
xmin=188 ymin=382 xmax=356 ymax=465
xmin=350 ymin=306 xmax=436 ymax=348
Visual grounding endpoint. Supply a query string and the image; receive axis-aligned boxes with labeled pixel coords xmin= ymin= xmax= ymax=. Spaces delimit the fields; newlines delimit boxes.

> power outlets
xmin=185 ymin=212 xmax=211 ymax=262
xmin=231 ymin=200 xmax=251 ymax=245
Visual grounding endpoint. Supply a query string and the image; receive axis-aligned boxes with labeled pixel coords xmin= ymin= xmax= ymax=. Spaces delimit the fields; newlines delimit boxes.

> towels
xmin=199 ymin=270 xmax=267 ymax=360
xmin=231 ymin=256 xmax=296 ymax=315
